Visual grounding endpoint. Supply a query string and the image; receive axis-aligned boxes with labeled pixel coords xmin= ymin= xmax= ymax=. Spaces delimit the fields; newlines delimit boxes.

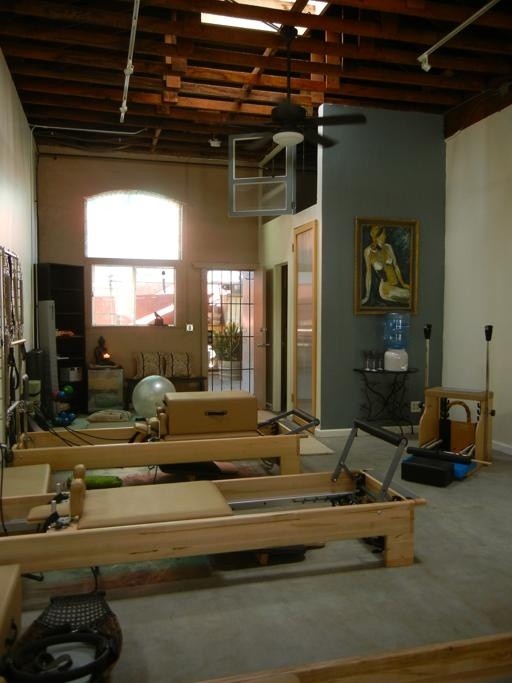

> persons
xmin=360 ymin=223 xmax=410 ymax=306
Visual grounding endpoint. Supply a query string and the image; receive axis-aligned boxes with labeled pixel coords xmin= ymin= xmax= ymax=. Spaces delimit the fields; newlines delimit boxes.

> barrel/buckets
xmin=382 ymin=313 xmax=410 ymax=348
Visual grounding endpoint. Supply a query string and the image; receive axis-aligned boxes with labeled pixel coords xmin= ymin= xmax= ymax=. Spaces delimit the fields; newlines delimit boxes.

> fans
xmin=226 ymin=24 xmax=367 ymax=148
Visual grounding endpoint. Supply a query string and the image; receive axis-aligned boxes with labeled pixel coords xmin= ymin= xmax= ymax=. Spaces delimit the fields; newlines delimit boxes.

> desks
xmin=420 ymin=387 xmax=494 ymax=465
xmin=353 ymin=368 xmax=418 ymax=436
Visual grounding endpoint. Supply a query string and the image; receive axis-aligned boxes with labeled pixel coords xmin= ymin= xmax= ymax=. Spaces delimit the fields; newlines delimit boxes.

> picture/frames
xmin=354 ymin=215 xmax=419 ymax=317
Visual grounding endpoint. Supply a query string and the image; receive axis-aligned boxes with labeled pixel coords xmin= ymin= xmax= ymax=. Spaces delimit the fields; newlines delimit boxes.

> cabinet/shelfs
xmin=0 ymin=564 xmax=23 ymax=666
xmin=36 ymin=262 xmax=87 ymax=413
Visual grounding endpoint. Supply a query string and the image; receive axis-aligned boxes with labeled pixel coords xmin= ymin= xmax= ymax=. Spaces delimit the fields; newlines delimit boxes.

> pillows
xmin=132 ymin=352 xmax=166 ymax=379
xmin=160 ymin=352 xmax=193 ymax=377
xmin=86 ymin=409 xmax=131 ymax=421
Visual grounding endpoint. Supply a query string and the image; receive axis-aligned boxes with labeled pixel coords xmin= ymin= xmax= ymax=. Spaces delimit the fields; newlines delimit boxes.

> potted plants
xmin=213 ymin=323 xmax=242 ymax=377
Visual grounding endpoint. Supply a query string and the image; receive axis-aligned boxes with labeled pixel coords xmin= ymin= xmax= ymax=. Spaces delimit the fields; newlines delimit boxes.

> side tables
xmin=88 ymin=369 xmax=124 ymax=413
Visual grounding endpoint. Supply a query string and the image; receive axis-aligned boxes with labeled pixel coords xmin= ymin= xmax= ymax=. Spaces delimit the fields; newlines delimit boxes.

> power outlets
xmin=411 ymin=401 xmax=421 ymax=413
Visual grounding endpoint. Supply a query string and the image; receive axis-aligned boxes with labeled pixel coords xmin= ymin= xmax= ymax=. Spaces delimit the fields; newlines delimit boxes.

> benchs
xmin=124 ymin=376 xmax=207 ymax=411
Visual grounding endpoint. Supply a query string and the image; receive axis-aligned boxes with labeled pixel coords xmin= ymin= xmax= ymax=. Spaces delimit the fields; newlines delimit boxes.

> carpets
xmin=258 ymin=410 xmax=334 ymax=455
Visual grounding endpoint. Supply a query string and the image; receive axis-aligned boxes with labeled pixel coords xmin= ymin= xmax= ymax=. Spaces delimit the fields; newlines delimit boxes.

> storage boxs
xmin=401 ymin=456 xmax=454 ymax=488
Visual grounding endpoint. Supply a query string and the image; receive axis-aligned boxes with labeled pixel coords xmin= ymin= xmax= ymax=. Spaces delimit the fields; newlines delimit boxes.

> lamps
xmin=119 ymin=0 xmax=141 ymax=123
xmin=417 ymin=0 xmax=499 ymax=72
